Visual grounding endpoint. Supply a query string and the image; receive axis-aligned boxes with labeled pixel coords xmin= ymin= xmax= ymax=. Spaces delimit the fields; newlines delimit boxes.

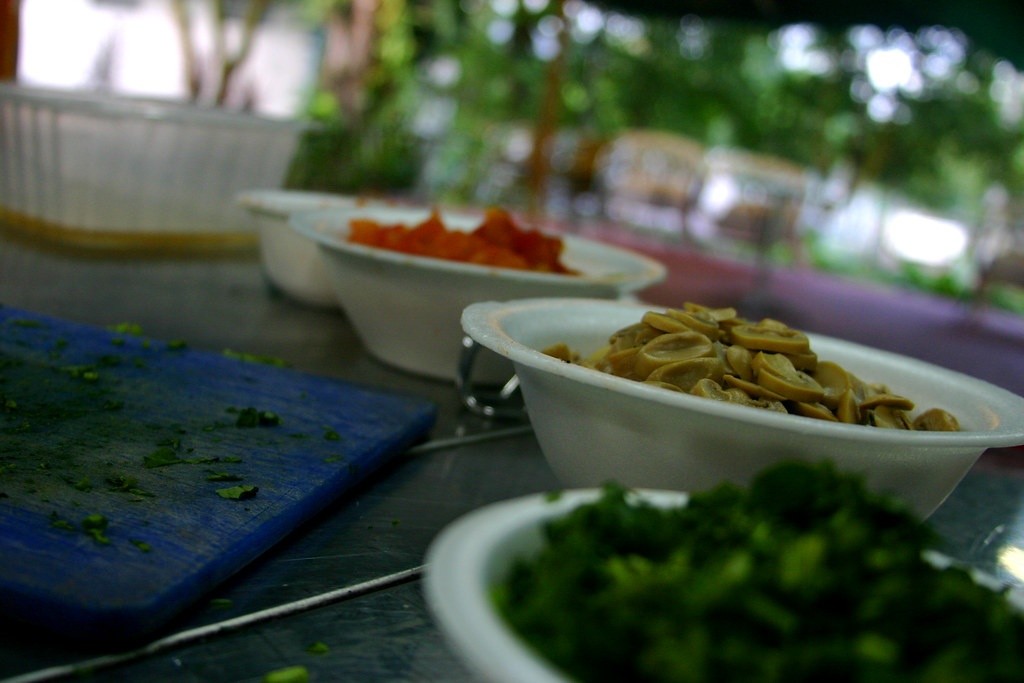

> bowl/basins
xmin=235 ymin=188 xmax=389 ymax=312
xmin=460 ymin=297 xmax=1024 ymax=523
xmin=284 ymin=206 xmax=668 ymax=387
xmin=0 ymin=83 xmax=309 ymax=259
xmin=422 ymin=487 xmax=1023 ymax=683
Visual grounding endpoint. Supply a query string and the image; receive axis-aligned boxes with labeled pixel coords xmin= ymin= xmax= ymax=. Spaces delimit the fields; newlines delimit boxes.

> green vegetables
xmin=485 ymin=455 xmax=1024 ymax=683
xmin=0 ymin=321 xmax=290 ymax=556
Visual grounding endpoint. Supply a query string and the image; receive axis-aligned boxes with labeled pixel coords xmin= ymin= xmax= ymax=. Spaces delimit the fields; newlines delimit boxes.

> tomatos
xmin=346 ymin=209 xmax=567 ymax=277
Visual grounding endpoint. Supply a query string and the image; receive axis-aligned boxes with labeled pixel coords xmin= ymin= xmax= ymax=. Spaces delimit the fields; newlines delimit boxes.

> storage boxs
xmin=0 ymin=81 xmax=311 ymax=265
